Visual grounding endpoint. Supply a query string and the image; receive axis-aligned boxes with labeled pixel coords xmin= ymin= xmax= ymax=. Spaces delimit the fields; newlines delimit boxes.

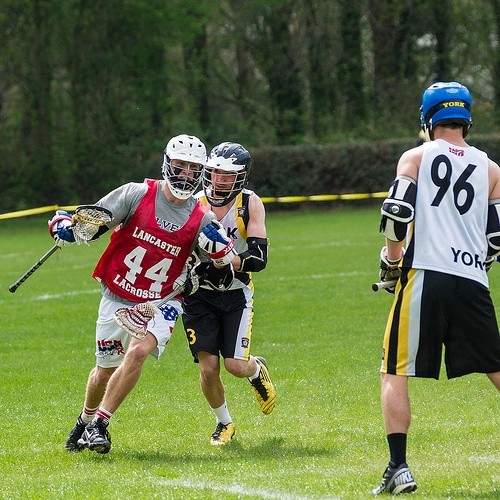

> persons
xmin=47 ymin=134 xmax=234 ymax=454
xmin=374 ymin=82 xmax=500 ymax=495
xmin=173 ymin=142 xmax=277 ymax=447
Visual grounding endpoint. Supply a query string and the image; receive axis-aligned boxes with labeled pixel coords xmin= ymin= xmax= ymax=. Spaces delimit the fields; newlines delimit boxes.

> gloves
xmin=484 ymin=256 xmax=495 ymax=272
xmin=48 ymin=210 xmax=78 ymax=247
xmin=199 ymin=219 xmax=239 ymax=267
xmin=379 ymin=246 xmax=406 ymax=294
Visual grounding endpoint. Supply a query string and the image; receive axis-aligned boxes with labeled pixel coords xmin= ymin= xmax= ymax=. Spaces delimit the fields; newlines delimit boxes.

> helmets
xmin=419 ymin=82 xmax=474 ymax=142
xmin=162 ymin=134 xmax=207 ymax=200
xmin=202 ymin=141 xmax=253 ymax=207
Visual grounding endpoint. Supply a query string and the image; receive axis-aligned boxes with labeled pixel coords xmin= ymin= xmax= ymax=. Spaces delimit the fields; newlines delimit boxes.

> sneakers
xmin=65 ymin=412 xmax=90 ymax=452
xmin=210 ymin=421 xmax=236 ymax=447
xmin=372 ymin=461 xmax=417 ymax=496
xmin=77 ymin=416 xmax=111 ymax=454
xmin=247 ymin=356 xmax=276 ymax=415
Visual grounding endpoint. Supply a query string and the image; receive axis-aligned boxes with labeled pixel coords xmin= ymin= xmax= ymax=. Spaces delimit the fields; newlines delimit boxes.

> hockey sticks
xmin=113 ymin=273 xmax=202 ymax=340
xmin=9 ymin=206 xmax=114 ymax=294
xmin=371 ymin=279 xmax=398 ymax=293
